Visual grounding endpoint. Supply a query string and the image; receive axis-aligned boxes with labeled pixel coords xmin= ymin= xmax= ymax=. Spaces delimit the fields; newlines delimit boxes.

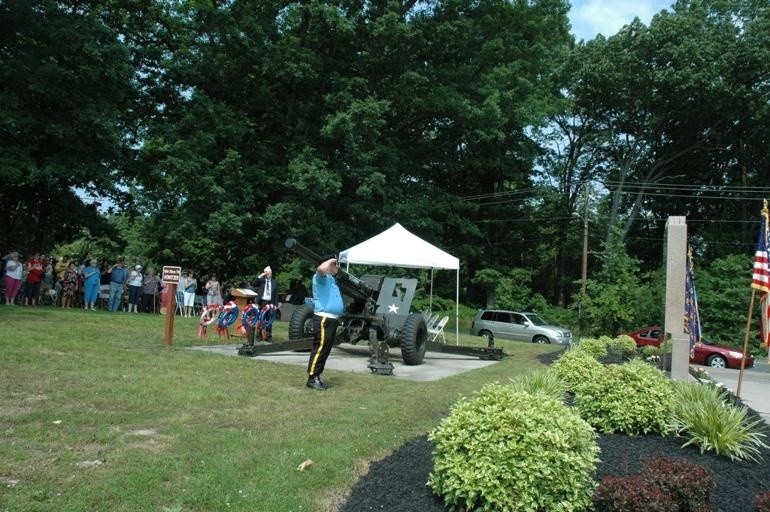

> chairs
xmin=421 ymin=309 xmax=450 ymax=343
xmin=0 ymin=269 xmax=210 ymax=316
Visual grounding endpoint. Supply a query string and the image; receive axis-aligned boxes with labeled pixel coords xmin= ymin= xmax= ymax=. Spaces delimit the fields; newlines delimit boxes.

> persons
xmin=184 ymin=269 xmax=198 ymax=318
xmin=205 ymin=273 xmax=222 ymax=317
xmin=0 ymin=245 xmax=169 ymax=314
xmin=306 ymin=253 xmax=344 ymax=391
xmin=252 ymin=266 xmax=279 ymax=342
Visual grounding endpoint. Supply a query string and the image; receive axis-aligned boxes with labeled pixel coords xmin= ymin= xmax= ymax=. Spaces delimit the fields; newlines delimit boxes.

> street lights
xmin=571 ymin=210 xmax=590 ymax=310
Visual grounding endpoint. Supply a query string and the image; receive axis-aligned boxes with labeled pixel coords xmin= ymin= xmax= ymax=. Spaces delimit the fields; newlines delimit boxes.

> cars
xmin=625 ymin=324 xmax=755 ymax=371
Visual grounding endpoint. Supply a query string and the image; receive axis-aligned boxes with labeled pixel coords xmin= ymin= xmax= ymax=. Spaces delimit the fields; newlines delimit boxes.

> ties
xmin=266 ymin=280 xmax=270 ymax=295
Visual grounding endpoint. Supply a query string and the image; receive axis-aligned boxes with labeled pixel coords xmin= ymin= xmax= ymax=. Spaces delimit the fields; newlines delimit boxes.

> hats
xmin=135 ymin=264 xmax=142 ymax=270
xmin=318 ymin=254 xmax=335 ymax=265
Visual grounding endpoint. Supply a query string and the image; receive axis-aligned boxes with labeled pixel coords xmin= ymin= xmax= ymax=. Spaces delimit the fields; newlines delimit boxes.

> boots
xmin=306 ymin=375 xmax=328 ymax=389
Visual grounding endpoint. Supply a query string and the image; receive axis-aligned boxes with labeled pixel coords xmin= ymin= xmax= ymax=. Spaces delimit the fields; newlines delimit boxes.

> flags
xmin=750 ymin=205 xmax=770 ymax=349
xmin=685 ymin=254 xmax=703 ymax=359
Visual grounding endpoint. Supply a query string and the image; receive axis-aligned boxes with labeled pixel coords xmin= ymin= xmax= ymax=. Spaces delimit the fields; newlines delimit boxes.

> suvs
xmin=467 ymin=307 xmax=573 ymax=347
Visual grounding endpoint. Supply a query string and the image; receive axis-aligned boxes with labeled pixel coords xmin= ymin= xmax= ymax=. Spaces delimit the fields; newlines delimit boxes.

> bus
xmin=97 ymin=271 xmax=112 ymax=299
